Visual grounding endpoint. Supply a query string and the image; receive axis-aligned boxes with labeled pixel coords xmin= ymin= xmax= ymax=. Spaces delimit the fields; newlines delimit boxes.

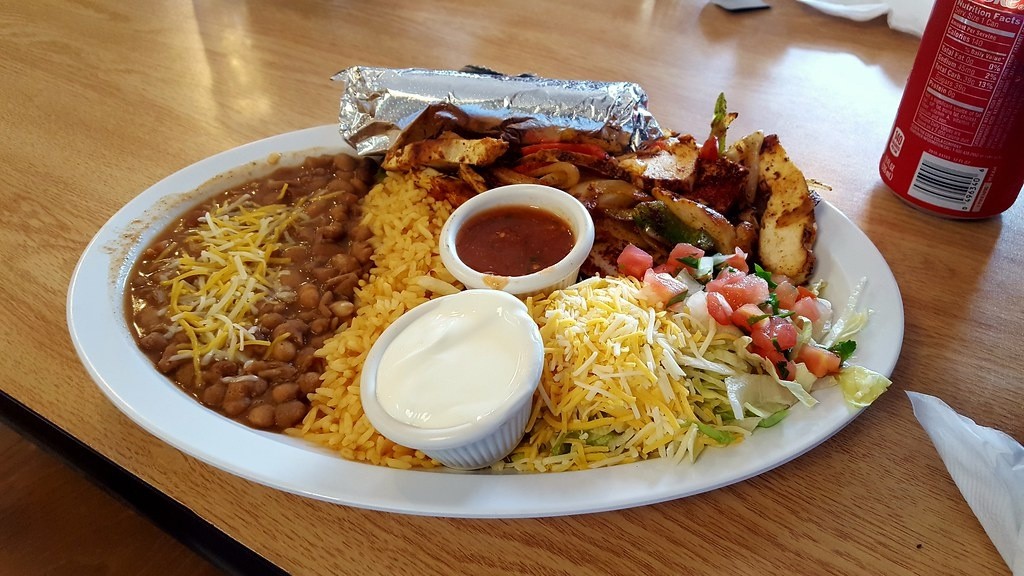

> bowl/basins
xmin=359 ymin=289 xmax=545 ymax=471
xmin=439 ymin=183 xmax=595 ymax=303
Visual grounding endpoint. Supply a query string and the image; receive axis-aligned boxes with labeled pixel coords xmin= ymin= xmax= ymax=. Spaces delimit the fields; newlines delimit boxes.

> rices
xmin=286 ymin=171 xmax=467 ymax=469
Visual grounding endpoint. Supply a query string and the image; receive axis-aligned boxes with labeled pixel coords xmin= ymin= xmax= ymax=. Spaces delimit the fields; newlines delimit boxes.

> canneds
xmin=878 ymin=0 xmax=1024 ymax=221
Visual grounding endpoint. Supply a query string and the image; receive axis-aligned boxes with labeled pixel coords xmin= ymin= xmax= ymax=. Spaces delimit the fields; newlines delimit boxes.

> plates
xmin=67 ymin=121 xmax=903 ymax=518
